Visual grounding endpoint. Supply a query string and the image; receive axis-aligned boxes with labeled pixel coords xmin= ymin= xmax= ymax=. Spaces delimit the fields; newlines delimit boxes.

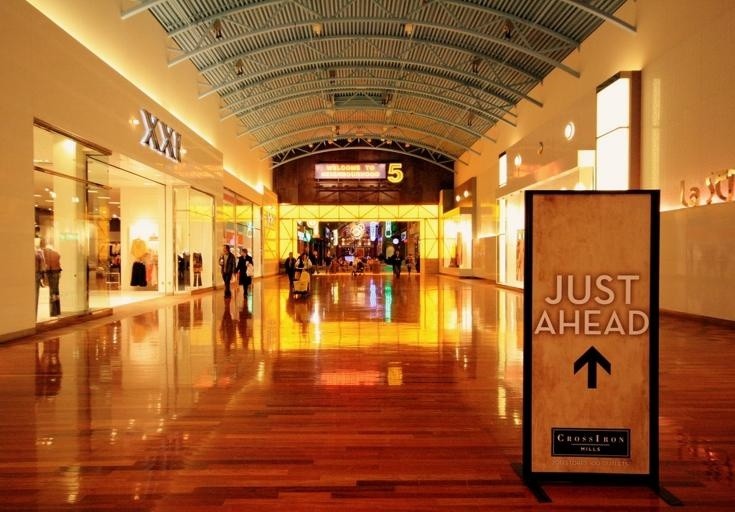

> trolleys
xmin=350 ymin=256 xmax=367 ymax=278
xmin=291 ymin=266 xmax=313 ymax=300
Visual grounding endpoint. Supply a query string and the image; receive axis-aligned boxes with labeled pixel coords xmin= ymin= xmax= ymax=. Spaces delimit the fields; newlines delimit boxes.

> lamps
xmin=514 ymin=155 xmax=522 ymax=167
xmin=563 ymin=122 xmax=575 ymax=140
xmin=456 ymin=191 xmax=470 ymax=201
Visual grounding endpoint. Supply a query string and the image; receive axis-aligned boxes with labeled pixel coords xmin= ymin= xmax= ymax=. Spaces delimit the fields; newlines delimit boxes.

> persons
xmin=219 ymin=297 xmax=236 ymax=358
xmin=233 ymin=247 xmax=254 ymax=300
xmin=38 ymin=237 xmax=62 ymax=318
xmin=35 ymin=250 xmax=45 ymax=321
xmin=285 ymin=293 xmax=316 ymax=339
xmin=220 ymin=244 xmax=238 ymax=300
xmin=235 ymin=301 xmax=255 ymax=352
xmin=40 ymin=337 xmax=64 ymax=406
xmin=34 ymin=342 xmax=45 ymax=406
xmin=282 ymin=246 xmax=420 ymax=294
